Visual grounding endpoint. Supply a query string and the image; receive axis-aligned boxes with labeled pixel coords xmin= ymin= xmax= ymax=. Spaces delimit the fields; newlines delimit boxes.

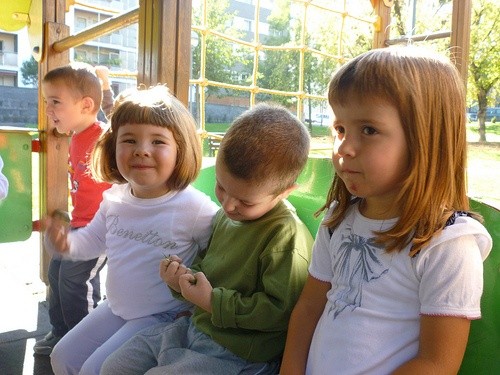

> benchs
xmin=187 ymin=158 xmax=500 ymax=375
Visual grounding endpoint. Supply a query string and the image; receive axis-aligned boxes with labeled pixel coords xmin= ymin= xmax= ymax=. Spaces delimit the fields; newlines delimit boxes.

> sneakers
xmin=33 ymin=331 xmax=62 ymax=355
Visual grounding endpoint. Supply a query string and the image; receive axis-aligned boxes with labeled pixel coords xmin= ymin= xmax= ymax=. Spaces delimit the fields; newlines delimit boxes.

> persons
xmin=279 ymin=45 xmax=493 ymax=375
xmin=33 ymin=62 xmax=315 ymax=375
